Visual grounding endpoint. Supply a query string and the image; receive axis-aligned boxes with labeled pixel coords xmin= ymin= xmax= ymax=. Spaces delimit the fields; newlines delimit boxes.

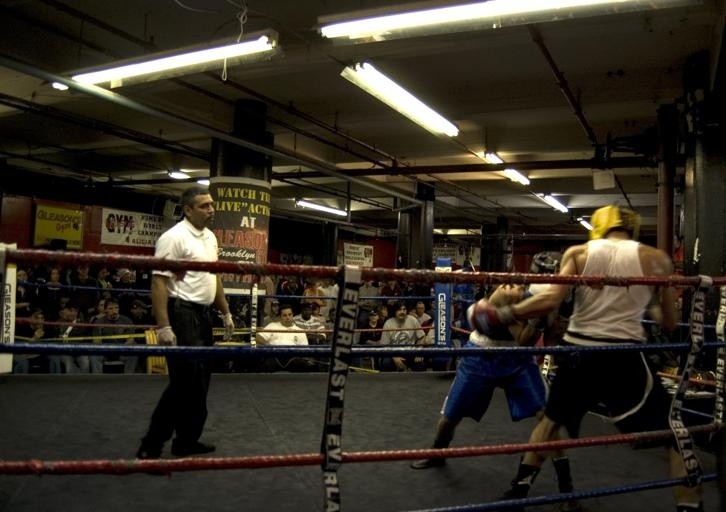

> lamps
xmin=51 ymin=1 xmax=284 ymax=91
xmin=475 ymin=128 xmax=531 ymax=186
xmin=576 ymin=217 xmax=593 ymax=231
xmin=543 ymin=192 xmax=569 ymax=213
xmin=295 ymin=196 xmax=347 ymax=217
xmin=316 ymin=0 xmax=630 ymax=46
xmin=339 ymin=59 xmax=459 ymax=139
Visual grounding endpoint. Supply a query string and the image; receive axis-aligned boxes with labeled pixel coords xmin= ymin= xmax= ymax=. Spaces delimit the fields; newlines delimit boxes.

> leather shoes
xmin=138 ymin=448 xmax=159 ymax=459
xmin=171 ymin=437 xmax=217 ymax=457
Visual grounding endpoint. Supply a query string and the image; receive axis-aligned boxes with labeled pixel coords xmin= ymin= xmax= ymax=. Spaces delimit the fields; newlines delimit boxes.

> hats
xmin=368 ymin=308 xmax=378 ymax=316
xmin=117 ymin=268 xmax=130 ymax=277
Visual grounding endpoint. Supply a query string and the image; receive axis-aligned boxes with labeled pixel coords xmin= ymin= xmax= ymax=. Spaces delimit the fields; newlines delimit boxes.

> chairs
xmin=144 ymin=330 xmax=169 ymax=376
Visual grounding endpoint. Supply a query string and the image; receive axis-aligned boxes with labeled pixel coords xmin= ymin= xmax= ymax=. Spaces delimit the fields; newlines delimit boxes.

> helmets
xmin=588 ymin=205 xmax=641 ymax=242
xmin=530 ymin=250 xmax=563 ymax=274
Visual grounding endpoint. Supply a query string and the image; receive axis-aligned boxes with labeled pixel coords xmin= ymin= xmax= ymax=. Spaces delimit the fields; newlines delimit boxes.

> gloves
xmin=155 ymin=326 xmax=177 ymax=346
xmin=466 ymin=298 xmax=520 ymax=334
xmin=221 ymin=311 xmax=235 ymax=342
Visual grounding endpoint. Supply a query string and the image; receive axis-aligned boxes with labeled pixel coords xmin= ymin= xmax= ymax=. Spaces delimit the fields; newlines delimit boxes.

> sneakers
xmin=558 ymin=502 xmax=584 ymax=511
xmin=410 ymin=445 xmax=448 ymax=470
xmin=676 ymin=500 xmax=706 ymax=512
